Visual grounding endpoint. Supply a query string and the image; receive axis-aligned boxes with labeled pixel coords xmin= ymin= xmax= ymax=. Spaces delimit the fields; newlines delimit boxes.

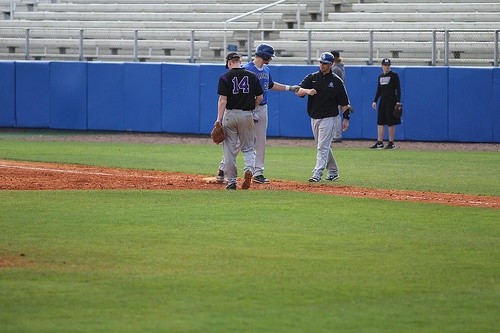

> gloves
xmin=289 ymin=86 xmax=301 ymax=93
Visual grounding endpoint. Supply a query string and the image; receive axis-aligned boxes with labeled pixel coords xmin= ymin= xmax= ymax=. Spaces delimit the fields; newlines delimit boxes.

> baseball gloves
xmin=211 ymin=121 xmax=226 ymax=145
xmin=393 ymin=104 xmax=402 ymax=119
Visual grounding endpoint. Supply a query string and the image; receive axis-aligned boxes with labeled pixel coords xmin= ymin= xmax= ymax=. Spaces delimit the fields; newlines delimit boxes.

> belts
xmin=226 ymin=108 xmax=250 ymax=112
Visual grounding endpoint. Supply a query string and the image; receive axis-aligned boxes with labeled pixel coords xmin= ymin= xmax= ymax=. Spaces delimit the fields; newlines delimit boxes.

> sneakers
xmin=323 ymin=175 xmax=339 ymax=181
xmin=217 ymin=170 xmax=224 ymax=182
xmin=385 ymin=142 xmax=396 ymax=149
xmin=253 ymin=175 xmax=270 ymax=184
xmin=308 ymin=175 xmax=321 ymax=182
xmin=370 ymin=143 xmax=384 ymax=149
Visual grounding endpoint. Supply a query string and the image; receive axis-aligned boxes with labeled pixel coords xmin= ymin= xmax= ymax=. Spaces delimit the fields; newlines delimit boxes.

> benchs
xmin=0 ymin=0 xmax=500 ymax=92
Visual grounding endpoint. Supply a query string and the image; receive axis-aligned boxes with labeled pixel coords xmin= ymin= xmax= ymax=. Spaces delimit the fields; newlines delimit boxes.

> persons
xmin=331 ymin=50 xmax=344 ymax=143
xmin=214 ymin=52 xmax=264 ymax=190
xmin=295 ymin=52 xmax=350 ymax=182
xmin=369 ymin=58 xmax=402 ymax=149
xmin=215 ymin=44 xmax=301 ymax=183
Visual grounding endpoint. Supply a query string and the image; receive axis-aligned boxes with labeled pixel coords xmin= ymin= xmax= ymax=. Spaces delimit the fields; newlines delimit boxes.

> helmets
xmin=255 ymin=43 xmax=276 ymax=61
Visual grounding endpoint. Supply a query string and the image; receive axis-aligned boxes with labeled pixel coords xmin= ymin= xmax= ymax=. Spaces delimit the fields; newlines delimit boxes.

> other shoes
xmin=332 ymin=138 xmax=342 ymax=143
xmin=226 ymin=183 xmax=236 ymax=190
xmin=241 ymin=170 xmax=252 ymax=189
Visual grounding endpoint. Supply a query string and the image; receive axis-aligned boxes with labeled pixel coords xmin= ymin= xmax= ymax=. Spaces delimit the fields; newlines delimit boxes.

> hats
xmin=317 ymin=52 xmax=335 ymax=64
xmin=331 ymin=51 xmax=340 ymax=57
xmin=225 ymin=53 xmax=240 ymax=69
xmin=382 ymin=59 xmax=391 ymax=65
